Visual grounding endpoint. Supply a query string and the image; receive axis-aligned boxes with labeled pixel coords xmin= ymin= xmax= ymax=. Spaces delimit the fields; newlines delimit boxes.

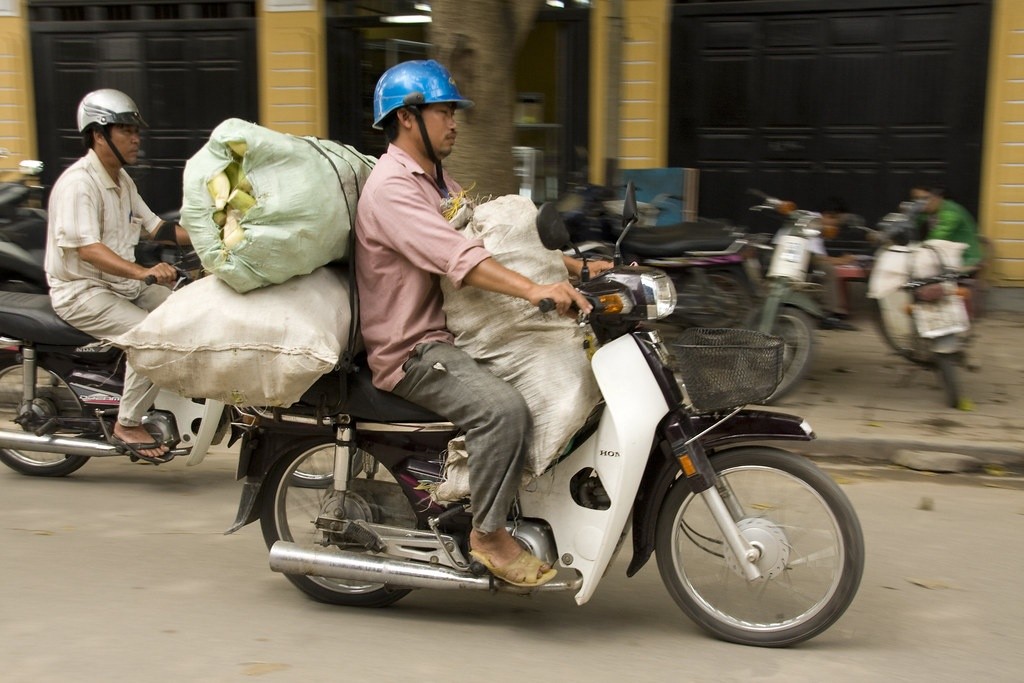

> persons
xmin=356 ymin=60 xmax=615 ymax=586
xmin=772 ymin=182 xmax=986 ymax=330
xmin=44 ymin=89 xmax=191 ymax=463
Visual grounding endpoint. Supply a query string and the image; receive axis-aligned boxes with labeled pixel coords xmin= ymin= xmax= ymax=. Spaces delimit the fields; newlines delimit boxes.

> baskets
xmin=666 ymin=325 xmax=785 ymax=416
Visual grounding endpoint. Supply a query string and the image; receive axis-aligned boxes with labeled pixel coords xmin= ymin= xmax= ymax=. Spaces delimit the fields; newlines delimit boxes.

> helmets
xmin=76 ymin=88 xmax=149 ymax=134
xmin=372 ymin=59 xmax=475 ymax=130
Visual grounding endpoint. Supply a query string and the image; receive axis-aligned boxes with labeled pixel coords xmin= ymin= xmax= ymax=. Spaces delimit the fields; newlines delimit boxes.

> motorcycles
xmin=0 ymin=157 xmax=367 ymax=491
xmin=557 ymin=184 xmax=870 ymax=406
xmin=218 ymin=182 xmax=867 ymax=650
xmin=822 ymin=197 xmax=982 ymax=410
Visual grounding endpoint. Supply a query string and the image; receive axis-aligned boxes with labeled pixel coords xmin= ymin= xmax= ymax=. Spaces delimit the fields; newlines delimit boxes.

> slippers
xmin=466 ymin=545 xmax=559 ymax=587
xmin=110 ymin=431 xmax=176 ymax=464
